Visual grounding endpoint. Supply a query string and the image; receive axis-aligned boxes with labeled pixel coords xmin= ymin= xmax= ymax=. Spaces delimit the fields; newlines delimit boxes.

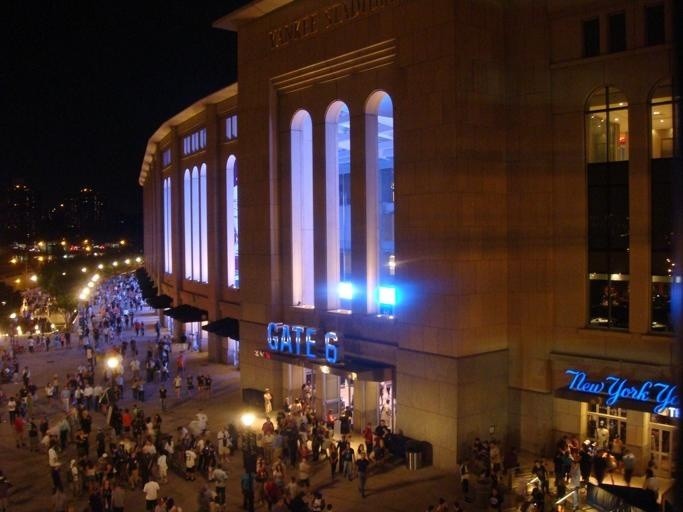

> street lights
xmin=8 ymin=312 xmax=16 ymax=346
xmin=108 ymin=358 xmax=120 ymax=436
xmin=79 ymin=274 xmax=99 ymax=336
xmin=242 ymin=414 xmax=255 ymax=512
